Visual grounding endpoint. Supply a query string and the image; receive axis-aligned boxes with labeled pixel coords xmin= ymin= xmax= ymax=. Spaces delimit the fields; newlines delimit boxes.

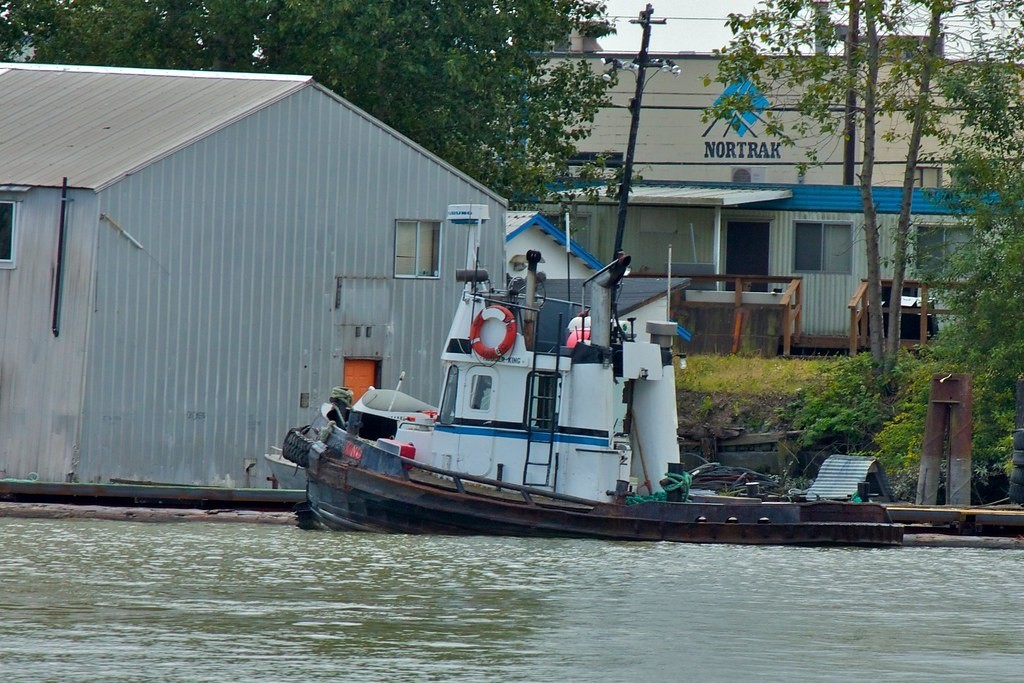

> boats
xmin=285 ymin=247 xmax=926 ymax=550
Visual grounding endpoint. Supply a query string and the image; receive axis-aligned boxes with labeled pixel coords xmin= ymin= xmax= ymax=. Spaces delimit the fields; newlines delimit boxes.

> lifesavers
xmin=469 ymin=304 xmax=516 ymax=360
